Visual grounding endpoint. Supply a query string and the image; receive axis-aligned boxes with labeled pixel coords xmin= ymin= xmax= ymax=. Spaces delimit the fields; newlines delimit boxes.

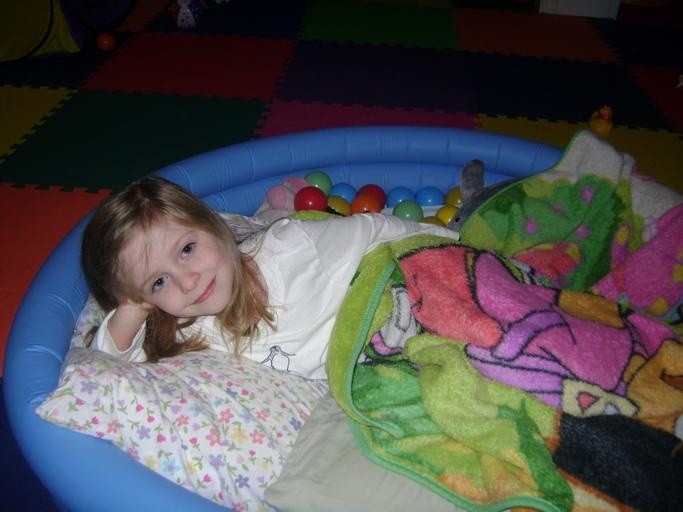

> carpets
xmin=0 ymin=0 xmax=683 ymax=510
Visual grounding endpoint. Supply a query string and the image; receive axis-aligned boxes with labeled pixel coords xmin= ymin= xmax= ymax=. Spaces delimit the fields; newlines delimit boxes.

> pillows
xmin=34 ymin=213 xmax=331 ymax=511
xmin=0 ymin=0 xmax=80 ymax=63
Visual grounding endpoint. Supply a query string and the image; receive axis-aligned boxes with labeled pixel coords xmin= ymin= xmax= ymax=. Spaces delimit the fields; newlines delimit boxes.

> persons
xmin=78 ymin=176 xmax=460 ymax=382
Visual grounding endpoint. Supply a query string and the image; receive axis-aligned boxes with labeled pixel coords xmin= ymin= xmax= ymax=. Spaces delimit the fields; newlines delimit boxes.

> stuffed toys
xmin=447 ymin=159 xmax=520 ymax=235
